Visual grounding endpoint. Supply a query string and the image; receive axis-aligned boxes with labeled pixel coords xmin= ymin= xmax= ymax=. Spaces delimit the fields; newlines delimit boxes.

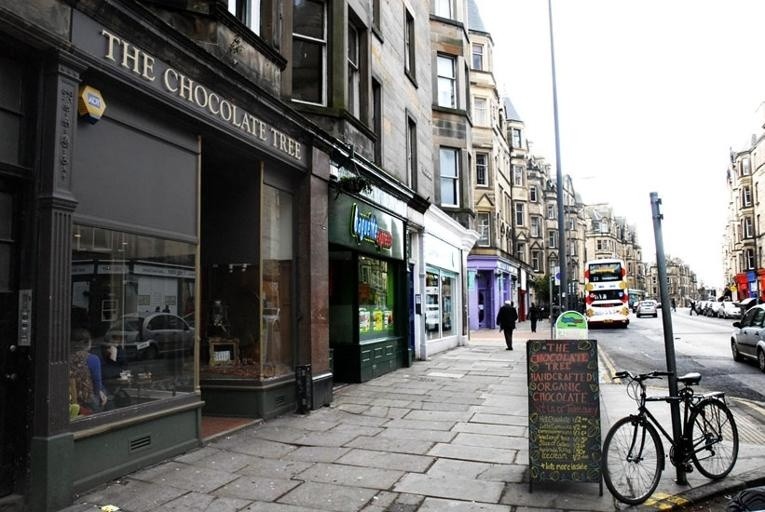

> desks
xmin=100 ymin=370 xmax=181 ymax=407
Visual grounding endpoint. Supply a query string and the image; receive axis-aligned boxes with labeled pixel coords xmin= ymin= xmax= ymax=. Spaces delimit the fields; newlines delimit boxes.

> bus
xmin=425 ymin=286 xmax=451 ymax=331
xmin=582 ymin=258 xmax=630 ymax=328
xmin=629 ymin=289 xmax=645 ymax=306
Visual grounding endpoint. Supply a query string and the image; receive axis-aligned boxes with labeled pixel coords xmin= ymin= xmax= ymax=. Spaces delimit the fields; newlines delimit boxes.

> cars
xmin=689 ymin=300 xmax=720 ymax=317
xmin=601 ymin=366 xmax=742 ymax=504
xmin=633 ymin=300 xmax=639 ymax=313
xmin=730 ymin=303 xmax=765 ymax=373
xmin=719 ymin=301 xmax=742 ymax=319
xmin=263 ymin=305 xmax=280 ymax=331
xmin=635 ymin=300 xmax=659 ymax=318
xmin=102 ymin=308 xmax=195 ymax=360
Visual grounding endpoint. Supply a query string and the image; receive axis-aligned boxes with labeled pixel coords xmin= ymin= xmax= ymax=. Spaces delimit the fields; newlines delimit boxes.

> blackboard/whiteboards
xmin=526 ymin=339 xmax=604 ymax=484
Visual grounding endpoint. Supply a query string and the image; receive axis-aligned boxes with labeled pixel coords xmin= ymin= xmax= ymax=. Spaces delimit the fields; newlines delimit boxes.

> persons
xmin=102 ymin=326 xmax=129 ymax=378
xmin=184 ymin=296 xmax=194 ymax=320
xmin=495 ymin=299 xmax=518 ymax=351
xmin=70 ymin=327 xmax=113 ymax=411
xmin=526 ymin=301 xmax=540 ymax=334
xmin=162 ymin=304 xmax=170 ymax=313
xmin=538 ymin=302 xmax=545 ymax=321
xmin=688 ymin=300 xmax=700 ymax=316
xmin=154 ymin=305 xmax=160 ymax=313
xmin=670 ymin=297 xmax=677 ymax=312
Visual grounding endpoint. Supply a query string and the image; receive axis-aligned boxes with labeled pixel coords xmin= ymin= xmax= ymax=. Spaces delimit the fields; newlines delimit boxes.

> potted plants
xmin=334 ymin=172 xmax=374 ymax=200
